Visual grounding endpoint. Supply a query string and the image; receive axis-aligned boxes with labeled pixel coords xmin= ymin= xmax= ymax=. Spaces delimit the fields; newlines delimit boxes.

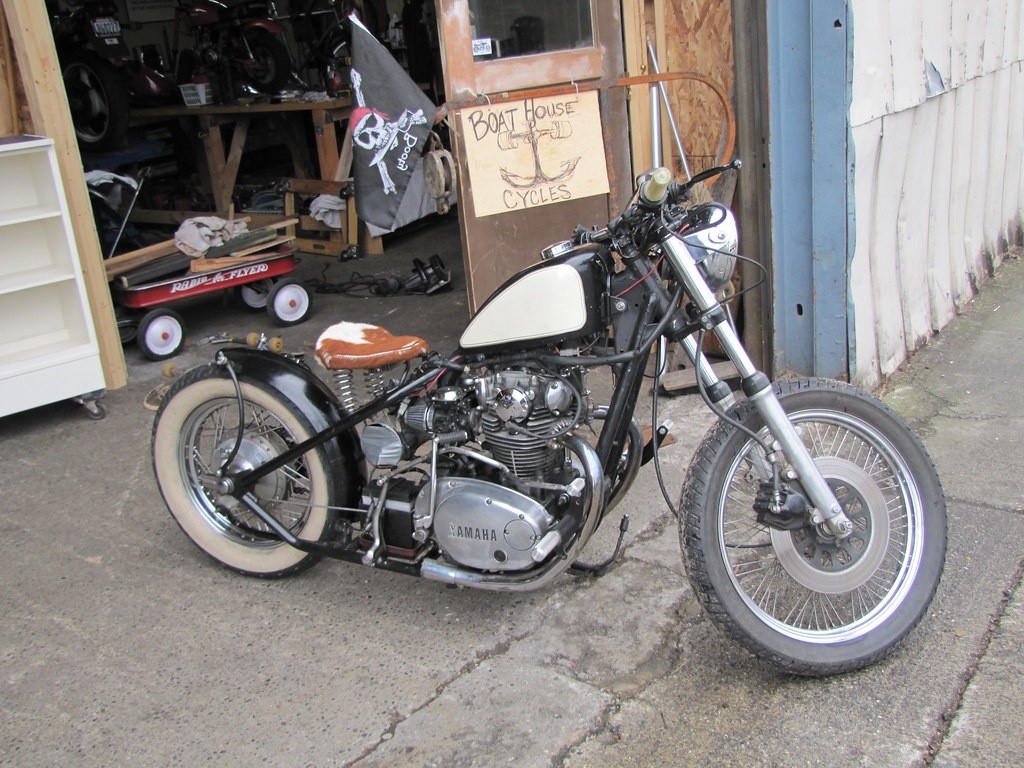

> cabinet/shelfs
xmin=0 ymin=135 xmax=107 ymax=421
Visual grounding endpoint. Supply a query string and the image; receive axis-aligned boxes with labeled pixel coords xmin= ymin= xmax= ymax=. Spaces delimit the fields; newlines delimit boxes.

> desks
xmin=120 ymin=96 xmax=355 ymax=253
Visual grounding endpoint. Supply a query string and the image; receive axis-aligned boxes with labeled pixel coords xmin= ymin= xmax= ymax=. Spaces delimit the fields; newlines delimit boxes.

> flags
xmin=348 ymin=13 xmax=440 ymax=230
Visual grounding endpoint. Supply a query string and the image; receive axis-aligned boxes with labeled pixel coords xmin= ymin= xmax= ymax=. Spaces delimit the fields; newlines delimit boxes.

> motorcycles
xmin=148 ymin=159 xmax=949 ymax=679
